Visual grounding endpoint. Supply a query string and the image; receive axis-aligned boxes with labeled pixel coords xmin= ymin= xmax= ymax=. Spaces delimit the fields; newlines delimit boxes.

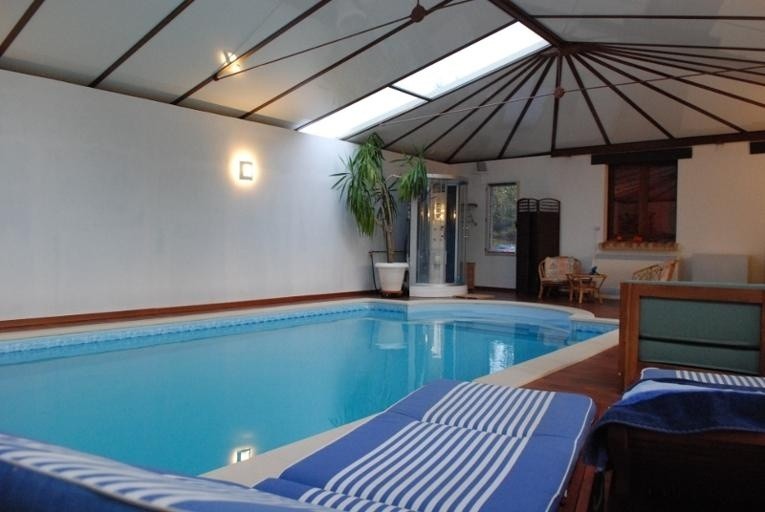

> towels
xmin=590 ymin=378 xmax=765 ymax=468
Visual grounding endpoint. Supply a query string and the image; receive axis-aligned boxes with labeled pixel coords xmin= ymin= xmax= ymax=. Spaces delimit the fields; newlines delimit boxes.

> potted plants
xmin=328 ymin=130 xmax=431 ymax=299
xmin=329 ymin=320 xmax=406 ymax=429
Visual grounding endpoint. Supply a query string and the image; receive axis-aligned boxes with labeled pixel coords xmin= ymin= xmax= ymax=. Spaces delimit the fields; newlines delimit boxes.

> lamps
xmin=240 ymin=162 xmax=254 ymax=181
xmin=237 ymin=449 xmax=251 ymax=465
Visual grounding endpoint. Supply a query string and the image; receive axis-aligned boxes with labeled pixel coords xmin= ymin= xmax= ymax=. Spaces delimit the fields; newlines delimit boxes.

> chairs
xmin=537 ymin=329 xmax=568 ymax=347
xmin=626 ymin=258 xmax=679 ymax=280
xmin=0 ymin=374 xmax=599 ymax=512
xmin=606 ymin=365 xmax=765 ymax=512
xmin=538 ymin=256 xmax=585 ymax=303
